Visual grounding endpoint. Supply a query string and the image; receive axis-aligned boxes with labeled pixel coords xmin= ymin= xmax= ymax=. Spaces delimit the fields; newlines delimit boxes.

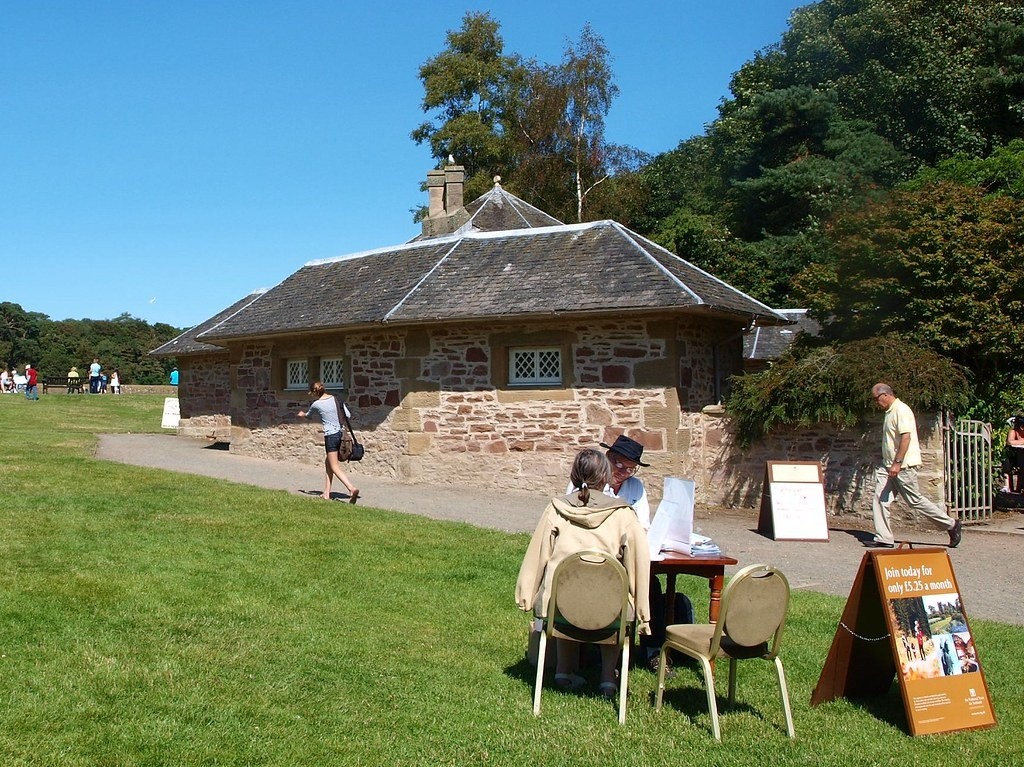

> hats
xmin=596 ymin=435 xmax=652 ymax=469
xmin=172 ymin=366 xmax=178 ymax=370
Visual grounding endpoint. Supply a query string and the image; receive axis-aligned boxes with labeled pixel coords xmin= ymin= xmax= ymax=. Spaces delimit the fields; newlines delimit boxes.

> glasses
xmin=874 ymin=393 xmax=884 ymax=401
xmin=611 ymin=457 xmax=635 ymax=475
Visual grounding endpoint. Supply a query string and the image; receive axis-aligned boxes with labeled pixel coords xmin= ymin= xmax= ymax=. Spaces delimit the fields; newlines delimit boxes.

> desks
xmin=650 ymin=555 xmax=739 ymax=687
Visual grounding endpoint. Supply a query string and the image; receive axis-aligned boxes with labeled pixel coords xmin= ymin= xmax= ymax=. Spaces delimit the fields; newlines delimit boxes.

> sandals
xmin=552 ymin=671 xmax=590 ymax=689
xmin=599 ymin=681 xmax=618 ymax=698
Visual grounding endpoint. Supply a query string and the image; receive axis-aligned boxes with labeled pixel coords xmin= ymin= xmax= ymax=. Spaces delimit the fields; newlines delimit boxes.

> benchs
xmin=41 ymin=375 xmax=89 ymax=394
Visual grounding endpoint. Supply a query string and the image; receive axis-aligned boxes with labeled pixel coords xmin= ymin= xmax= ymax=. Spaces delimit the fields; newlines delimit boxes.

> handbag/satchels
xmin=351 ymin=443 xmax=365 ymax=461
xmin=338 ymin=432 xmax=353 ymax=463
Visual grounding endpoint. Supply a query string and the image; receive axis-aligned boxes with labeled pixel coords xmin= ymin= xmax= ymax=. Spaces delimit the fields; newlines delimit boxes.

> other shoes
xmin=347 ymin=489 xmax=361 ymax=507
xmin=946 ymin=519 xmax=964 ymax=549
xmin=648 ymin=651 xmax=677 ymax=677
xmin=317 ymin=494 xmax=330 ymax=499
xmin=862 ymin=539 xmax=896 ymax=549
xmin=999 ymin=487 xmax=1012 ymax=493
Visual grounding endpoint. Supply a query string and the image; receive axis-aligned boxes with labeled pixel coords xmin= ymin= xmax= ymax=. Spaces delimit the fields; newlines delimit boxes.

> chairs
xmin=654 ymin=562 xmax=795 ymax=741
xmin=533 ymin=547 xmax=630 ymax=725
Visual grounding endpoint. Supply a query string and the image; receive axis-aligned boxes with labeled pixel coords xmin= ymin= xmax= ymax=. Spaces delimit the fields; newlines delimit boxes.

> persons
xmin=1004 ymin=414 xmax=1024 ymax=492
xmin=0 ymin=367 xmax=18 ymax=393
xmin=87 ymin=358 xmax=110 ymax=394
xmin=514 ymin=434 xmax=676 ymax=696
xmin=862 ymin=383 xmax=961 ymax=547
xmin=24 ymin=364 xmax=41 ymax=401
xmin=297 ymin=381 xmax=359 ymax=504
xmin=169 ymin=367 xmax=178 ymax=386
xmin=67 ymin=366 xmax=80 ymax=394
xmin=901 ymin=621 xmax=926 ymax=661
xmin=110 ymin=368 xmax=121 ymax=394
